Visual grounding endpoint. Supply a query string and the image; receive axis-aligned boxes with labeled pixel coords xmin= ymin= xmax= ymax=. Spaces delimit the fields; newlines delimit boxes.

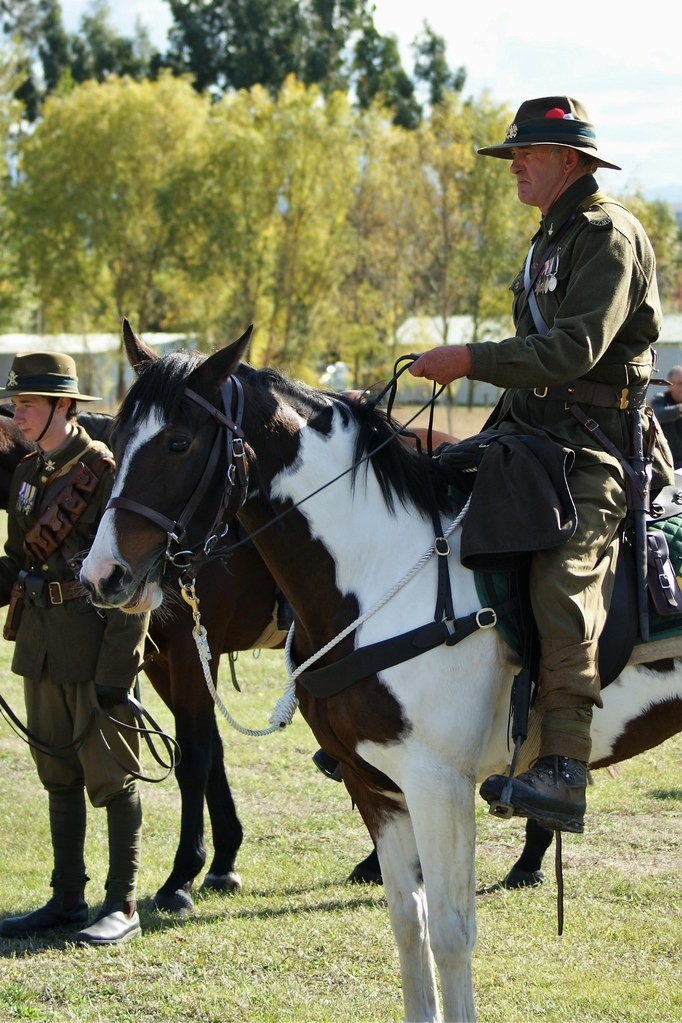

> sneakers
xmin=313 ymin=748 xmax=343 ymax=780
xmin=480 ymin=756 xmax=587 ymax=833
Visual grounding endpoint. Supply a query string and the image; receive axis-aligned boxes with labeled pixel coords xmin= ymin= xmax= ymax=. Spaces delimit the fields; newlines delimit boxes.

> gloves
xmin=95 ymin=683 xmax=128 ymax=709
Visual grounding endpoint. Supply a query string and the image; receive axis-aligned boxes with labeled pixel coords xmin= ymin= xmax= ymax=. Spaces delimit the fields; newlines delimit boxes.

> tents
xmin=0 ymin=331 xmax=198 ymax=405
xmin=384 ymin=317 xmax=681 ymax=407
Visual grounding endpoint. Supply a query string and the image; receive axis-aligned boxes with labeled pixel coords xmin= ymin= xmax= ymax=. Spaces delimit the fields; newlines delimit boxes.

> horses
xmin=0 ymin=317 xmax=681 ymax=1023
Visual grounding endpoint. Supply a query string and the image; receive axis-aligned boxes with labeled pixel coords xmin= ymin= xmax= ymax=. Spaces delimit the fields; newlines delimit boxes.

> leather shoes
xmin=0 ymin=891 xmax=88 ymax=937
xmin=78 ymin=901 xmax=141 ymax=944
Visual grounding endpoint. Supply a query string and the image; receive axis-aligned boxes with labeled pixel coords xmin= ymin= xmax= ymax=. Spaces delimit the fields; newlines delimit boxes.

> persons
xmin=1 ymin=352 xmax=150 ymax=947
xmin=650 ymin=365 xmax=681 ymax=470
xmin=318 ymin=351 xmax=347 ymax=391
xmin=404 ymin=96 xmax=663 ymax=832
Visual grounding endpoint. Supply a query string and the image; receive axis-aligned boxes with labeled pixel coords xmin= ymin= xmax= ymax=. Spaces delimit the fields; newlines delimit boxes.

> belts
xmin=534 ymin=379 xmax=649 ymax=409
xmin=48 ymin=581 xmax=90 ymax=604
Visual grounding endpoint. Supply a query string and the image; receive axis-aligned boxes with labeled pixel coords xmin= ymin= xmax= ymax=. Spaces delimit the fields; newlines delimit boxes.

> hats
xmin=0 ymin=351 xmax=103 ymax=402
xmin=477 ymin=96 xmax=622 ymax=171
